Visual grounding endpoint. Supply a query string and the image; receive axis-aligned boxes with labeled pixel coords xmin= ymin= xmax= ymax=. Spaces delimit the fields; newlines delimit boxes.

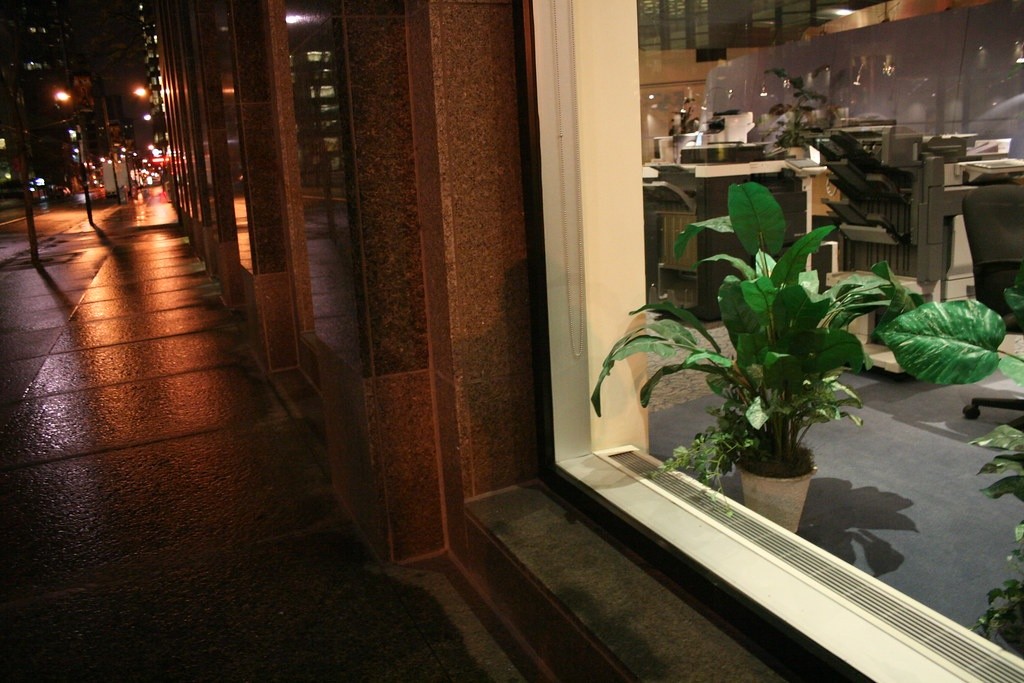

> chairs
xmin=961 ymin=182 xmax=1024 ymax=432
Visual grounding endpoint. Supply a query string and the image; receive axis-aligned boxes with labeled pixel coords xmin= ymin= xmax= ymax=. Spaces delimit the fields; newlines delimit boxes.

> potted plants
xmin=760 ymin=61 xmax=837 ymax=161
xmin=589 ymin=181 xmax=925 ymax=534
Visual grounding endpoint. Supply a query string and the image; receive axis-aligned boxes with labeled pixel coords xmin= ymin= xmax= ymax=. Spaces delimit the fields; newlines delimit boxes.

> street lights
xmin=50 ymin=0 xmax=93 ymax=223
